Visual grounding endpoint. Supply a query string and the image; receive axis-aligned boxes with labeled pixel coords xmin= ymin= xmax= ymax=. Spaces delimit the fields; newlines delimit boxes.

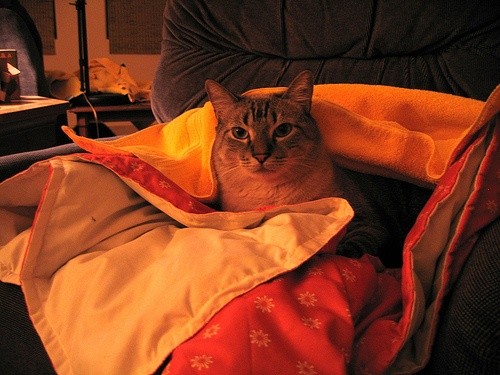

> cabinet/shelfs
xmin=0 ymin=95 xmax=70 ymax=156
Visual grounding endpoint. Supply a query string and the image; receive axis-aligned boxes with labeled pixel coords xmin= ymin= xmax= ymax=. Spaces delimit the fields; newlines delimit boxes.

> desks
xmin=68 ymin=103 xmax=152 ymax=139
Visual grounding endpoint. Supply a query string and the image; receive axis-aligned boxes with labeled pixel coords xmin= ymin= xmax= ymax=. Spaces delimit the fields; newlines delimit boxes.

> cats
xmin=204 ymin=70 xmax=401 ymax=267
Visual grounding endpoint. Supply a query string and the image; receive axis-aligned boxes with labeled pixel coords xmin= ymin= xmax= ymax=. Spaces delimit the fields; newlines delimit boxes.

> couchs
xmin=0 ymin=0 xmax=500 ymax=375
